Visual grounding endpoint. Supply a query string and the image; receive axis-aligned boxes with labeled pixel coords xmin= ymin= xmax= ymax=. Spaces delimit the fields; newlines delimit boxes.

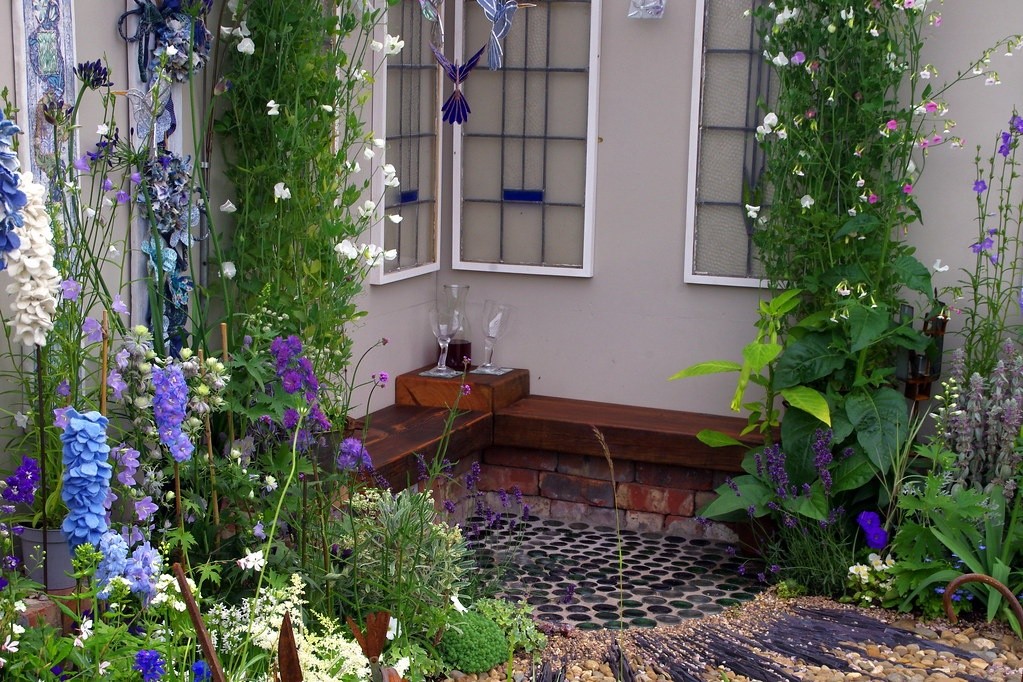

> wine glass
xmin=476 ymin=299 xmax=513 ymax=375
xmin=424 ymin=299 xmax=463 ymax=374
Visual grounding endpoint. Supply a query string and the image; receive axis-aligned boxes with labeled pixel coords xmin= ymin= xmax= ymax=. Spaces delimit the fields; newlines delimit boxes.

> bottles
xmin=437 ymin=284 xmax=471 ymax=370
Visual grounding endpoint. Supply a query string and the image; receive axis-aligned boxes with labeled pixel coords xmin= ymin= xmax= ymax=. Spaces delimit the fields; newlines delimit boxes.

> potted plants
xmin=0 ymin=300 xmax=94 ymax=590
